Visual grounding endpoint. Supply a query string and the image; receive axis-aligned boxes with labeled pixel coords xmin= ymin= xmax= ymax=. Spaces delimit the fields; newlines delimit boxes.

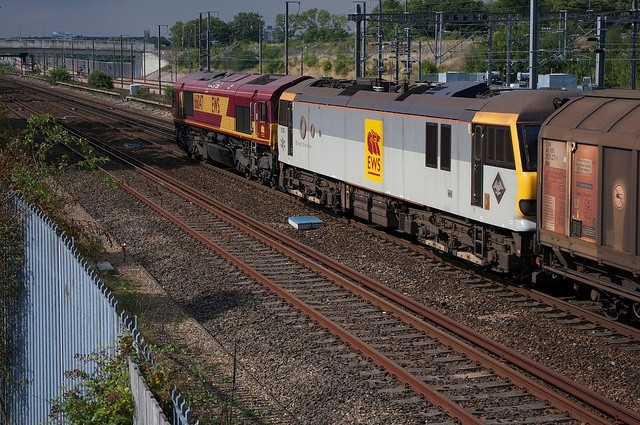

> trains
xmin=173 ymin=68 xmax=638 ymax=318
